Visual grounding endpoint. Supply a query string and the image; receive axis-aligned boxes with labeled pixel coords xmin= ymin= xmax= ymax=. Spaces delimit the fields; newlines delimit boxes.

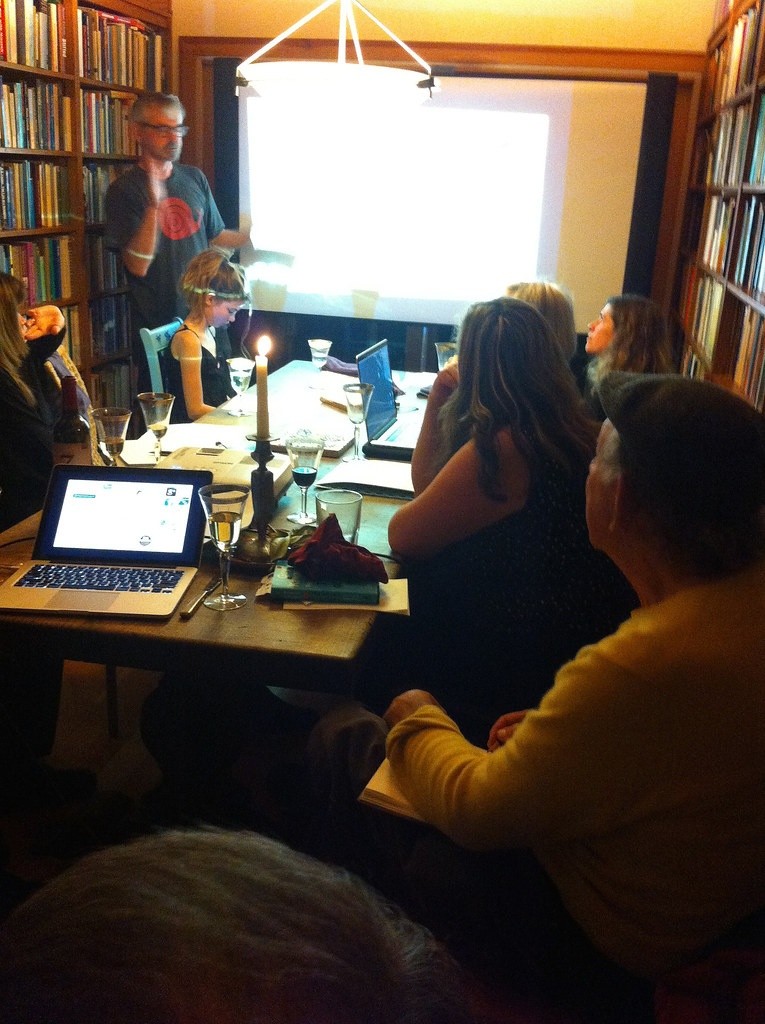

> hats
xmin=599 ymin=369 xmax=765 ymax=545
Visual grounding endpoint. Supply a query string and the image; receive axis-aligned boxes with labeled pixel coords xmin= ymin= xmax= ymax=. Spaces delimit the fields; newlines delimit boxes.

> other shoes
xmin=143 ymin=787 xmax=246 ymax=833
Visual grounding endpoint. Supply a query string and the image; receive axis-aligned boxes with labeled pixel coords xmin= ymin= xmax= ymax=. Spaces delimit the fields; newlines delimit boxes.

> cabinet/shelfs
xmin=668 ymin=0 xmax=765 ymax=410
xmin=0 ymin=0 xmax=174 ymax=439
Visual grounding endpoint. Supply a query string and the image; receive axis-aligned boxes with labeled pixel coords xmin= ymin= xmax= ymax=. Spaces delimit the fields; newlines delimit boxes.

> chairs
xmin=140 ymin=315 xmax=183 ymax=424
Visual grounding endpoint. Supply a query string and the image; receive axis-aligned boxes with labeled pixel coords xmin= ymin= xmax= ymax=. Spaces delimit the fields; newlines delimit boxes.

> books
xmin=271 ymin=430 xmax=355 ymax=458
xmin=1 ymin=0 xmax=163 ymax=436
xmin=271 ymin=560 xmax=380 ymax=606
xmin=678 ymin=0 xmax=764 ymax=407
xmin=148 ymin=447 xmax=293 ymax=546
xmin=356 ymin=756 xmax=431 ymax=824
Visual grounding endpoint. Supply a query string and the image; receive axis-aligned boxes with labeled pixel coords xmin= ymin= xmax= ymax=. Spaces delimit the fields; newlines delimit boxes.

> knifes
xmin=179 ymin=572 xmax=222 ymax=619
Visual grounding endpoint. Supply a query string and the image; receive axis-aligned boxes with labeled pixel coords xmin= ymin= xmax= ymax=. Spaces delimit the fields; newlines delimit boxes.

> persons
xmin=0 ymin=828 xmax=473 ymax=1024
xmin=506 ymin=284 xmax=575 ymax=364
xmin=0 ymin=272 xmax=105 ymax=818
xmin=105 ymin=93 xmax=249 ymax=394
xmin=577 ymin=294 xmax=671 ymax=423
xmin=358 ymin=295 xmax=637 ymax=734
xmin=165 ymin=248 xmax=249 ymax=423
xmin=302 ymin=369 xmax=765 ymax=1023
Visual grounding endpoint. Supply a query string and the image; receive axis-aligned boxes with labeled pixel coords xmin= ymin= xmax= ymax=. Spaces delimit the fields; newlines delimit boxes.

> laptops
xmin=356 ymin=337 xmax=425 ymax=448
xmin=0 ymin=464 xmax=214 ymax=619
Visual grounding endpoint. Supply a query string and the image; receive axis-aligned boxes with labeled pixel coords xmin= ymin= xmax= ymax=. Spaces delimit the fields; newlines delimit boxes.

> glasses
xmin=136 ymin=119 xmax=188 ymax=138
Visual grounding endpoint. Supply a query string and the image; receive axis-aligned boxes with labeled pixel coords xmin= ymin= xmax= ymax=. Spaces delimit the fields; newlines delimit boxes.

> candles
xmin=254 ymin=335 xmax=272 ymax=437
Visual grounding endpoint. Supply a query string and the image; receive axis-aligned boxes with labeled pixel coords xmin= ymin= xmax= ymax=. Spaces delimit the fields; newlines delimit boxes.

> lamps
xmin=237 ymin=0 xmax=439 ymax=123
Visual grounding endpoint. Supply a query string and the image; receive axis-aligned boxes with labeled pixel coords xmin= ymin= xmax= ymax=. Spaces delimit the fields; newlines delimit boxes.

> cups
xmin=435 ymin=342 xmax=459 ymax=371
xmin=308 ymin=339 xmax=332 ymax=368
xmin=315 ymin=489 xmax=363 ymax=546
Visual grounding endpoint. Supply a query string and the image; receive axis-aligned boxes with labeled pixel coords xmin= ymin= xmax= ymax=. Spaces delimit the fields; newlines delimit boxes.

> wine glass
xmin=286 ymin=434 xmax=326 ymax=525
xmin=137 ymin=392 xmax=175 ymax=465
xmin=342 ymin=382 xmax=376 ymax=463
xmin=198 ymin=483 xmax=251 ymax=610
xmin=92 ymin=406 xmax=132 ymax=466
xmin=226 ymin=357 xmax=255 ymax=417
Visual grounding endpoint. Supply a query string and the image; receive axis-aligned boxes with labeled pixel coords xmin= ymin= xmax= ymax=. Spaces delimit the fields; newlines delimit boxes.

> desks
xmin=0 ymin=360 xmax=439 ymax=735
